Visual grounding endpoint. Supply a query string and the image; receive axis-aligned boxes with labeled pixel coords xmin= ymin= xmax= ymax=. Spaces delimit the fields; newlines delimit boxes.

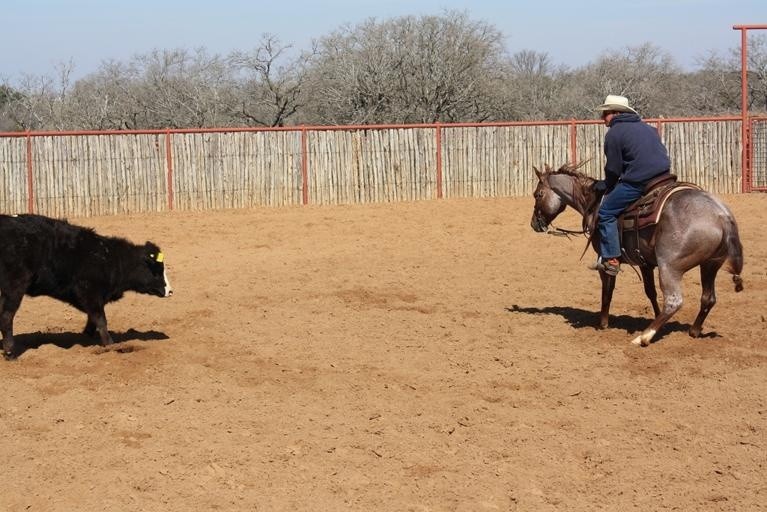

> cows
xmin=0 ymin=211 xmax=173 ymax=361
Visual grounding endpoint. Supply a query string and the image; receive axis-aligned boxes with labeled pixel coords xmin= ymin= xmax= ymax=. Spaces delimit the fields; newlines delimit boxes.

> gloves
xmin=592 ymin=180 xmax=609 ymax=192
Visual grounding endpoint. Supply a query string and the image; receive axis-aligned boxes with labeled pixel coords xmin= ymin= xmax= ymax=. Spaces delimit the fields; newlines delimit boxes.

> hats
xmin=594 ymin=94 xmax=638 ymax=114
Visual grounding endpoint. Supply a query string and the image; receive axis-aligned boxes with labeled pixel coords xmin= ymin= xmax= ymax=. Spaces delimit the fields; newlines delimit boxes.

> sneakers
xmin=588 ymin=258 xmax=620 ymax=276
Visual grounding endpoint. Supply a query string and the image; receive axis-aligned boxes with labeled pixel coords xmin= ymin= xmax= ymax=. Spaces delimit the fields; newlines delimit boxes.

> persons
xmin=587 ymin=94 xmax=671 ymax=276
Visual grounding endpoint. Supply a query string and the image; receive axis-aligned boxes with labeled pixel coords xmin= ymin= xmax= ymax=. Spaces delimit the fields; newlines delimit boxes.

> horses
xmin=531 ymin=158 xmax=743 ymax=347
